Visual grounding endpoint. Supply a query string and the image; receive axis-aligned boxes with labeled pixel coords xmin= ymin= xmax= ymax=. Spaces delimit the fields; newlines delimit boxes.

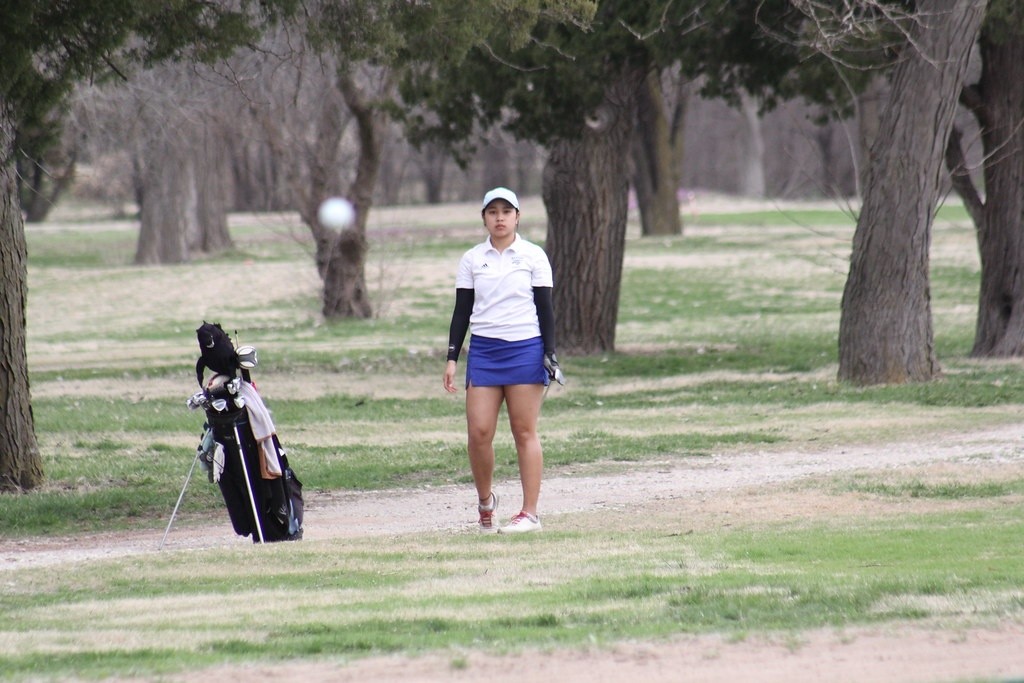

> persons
xmin=442 ymin=185 xmax=567 ymax=537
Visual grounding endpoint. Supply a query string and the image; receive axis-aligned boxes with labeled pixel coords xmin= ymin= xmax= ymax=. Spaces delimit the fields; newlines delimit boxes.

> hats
xmin=483 ymin=186 xmax=521 ymax=213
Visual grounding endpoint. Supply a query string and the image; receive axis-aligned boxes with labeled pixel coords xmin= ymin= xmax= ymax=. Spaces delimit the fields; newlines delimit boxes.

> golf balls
xmin=317 ymin=196 xmax=358 ymax=231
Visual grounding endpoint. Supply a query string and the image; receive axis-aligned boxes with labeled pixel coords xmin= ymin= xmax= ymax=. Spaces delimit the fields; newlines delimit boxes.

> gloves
xmin=545 ymin=352 xmax=565 ymax=385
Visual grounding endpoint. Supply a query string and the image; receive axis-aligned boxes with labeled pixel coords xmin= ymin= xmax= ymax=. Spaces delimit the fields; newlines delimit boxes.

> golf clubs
xmin=544 ymin=368 xmax=566 ymax=402
xmin=185 ymin=376 xmax=245 ymax=413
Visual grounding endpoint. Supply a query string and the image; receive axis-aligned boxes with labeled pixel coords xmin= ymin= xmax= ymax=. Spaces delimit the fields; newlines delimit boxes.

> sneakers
xmin=498 ymin=510 xmax=542 ymax=535
xmin=478 ymin=491 xmax=498 ymax=536
